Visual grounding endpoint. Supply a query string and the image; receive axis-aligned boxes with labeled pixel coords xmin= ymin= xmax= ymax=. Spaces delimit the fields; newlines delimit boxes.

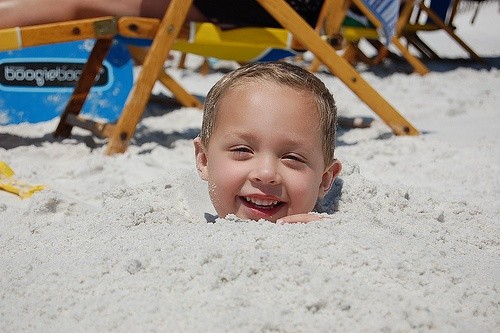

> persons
xmin=1 ymin=0 xmax=325 ymax=32
xmin=193 ymin=61 xmax=341 ymax=224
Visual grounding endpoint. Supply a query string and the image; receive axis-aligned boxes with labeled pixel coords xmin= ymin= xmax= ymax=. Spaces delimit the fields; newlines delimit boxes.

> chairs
xmin=0 ymin=1 xmax=490 ymax=203
